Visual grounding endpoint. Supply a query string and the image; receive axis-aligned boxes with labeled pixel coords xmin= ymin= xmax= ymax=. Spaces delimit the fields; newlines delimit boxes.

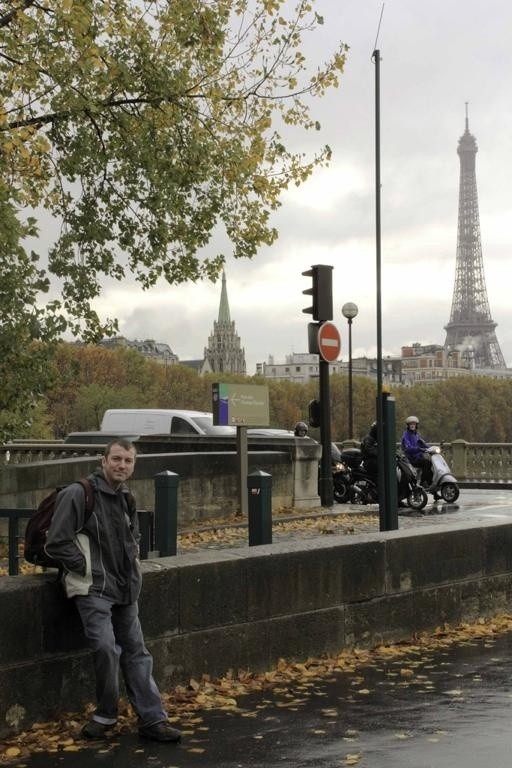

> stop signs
xmin=317 ymin=322 xmax=342 ymax=362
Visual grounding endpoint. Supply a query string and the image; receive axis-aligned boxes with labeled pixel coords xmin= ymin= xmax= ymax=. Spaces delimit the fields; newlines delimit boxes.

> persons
xmin=41 ymin=436 xmax=183 ymax=745
xmin=398 ymin=414 xmax=444 ymax=501
xmin=362 ymin=414 xmax=410 ymax=508
xmin=293 ymin=422 xmax=310 ymax=436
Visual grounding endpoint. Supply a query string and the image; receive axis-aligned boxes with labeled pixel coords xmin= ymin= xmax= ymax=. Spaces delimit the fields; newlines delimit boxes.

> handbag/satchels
xmin=23 ymin=477 xmax=95 ymax=568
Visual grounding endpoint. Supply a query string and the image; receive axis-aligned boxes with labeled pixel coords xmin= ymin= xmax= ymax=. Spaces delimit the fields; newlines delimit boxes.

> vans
xmin=101 ymin=407 xmax=238 ymax=436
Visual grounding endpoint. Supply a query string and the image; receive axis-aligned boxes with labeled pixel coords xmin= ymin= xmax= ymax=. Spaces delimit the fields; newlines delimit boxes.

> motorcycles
xmin=407 ymin=439 xmax=460 ymax=502
xmin=317 ymin=442 xmax=351 ymax=502
xmin=340 ymin=447 xmax=428 ymax=509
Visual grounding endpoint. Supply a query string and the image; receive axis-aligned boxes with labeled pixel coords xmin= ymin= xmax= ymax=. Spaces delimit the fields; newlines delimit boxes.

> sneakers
xmin=137 ymin=722 xmax=181 ymax=742
xmin=81 ymin=720 xmax=118 ymax=737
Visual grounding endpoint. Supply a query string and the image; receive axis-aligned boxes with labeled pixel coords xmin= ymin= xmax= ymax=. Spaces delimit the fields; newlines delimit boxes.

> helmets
xmin=295 ymin=422 xmax=308 ymax=435
xmin=405 ymin=415 xmax=420 ymax=430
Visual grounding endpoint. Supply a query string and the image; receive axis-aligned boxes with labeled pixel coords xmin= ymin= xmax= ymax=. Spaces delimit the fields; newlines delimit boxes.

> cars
xmin=245 ymin=428 xmax=320 ymax=444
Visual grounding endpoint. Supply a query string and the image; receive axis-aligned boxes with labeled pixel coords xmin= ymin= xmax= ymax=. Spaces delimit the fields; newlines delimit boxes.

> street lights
xmin=341 ymin=300 xmax=359 ymax=439
